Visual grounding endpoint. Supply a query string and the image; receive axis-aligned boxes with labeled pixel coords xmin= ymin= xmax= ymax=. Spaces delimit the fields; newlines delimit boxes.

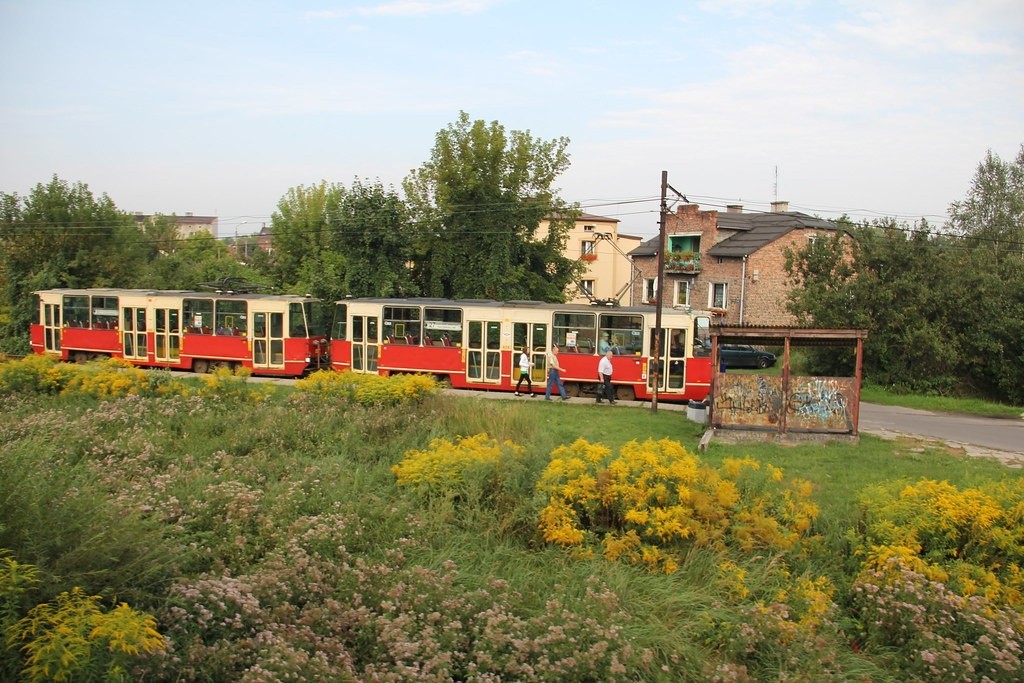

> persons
xmin=221 ymin=322 xmax=233 ymax=336
xmin=514 ymin=346 xmax=537 ymax=398
xmin=611 ymin=335 xmax=627 ymax=355
xmin=545 ymin=346 xmax=571 ymax=400
xmin=598 ymin=331 xmax=617 ymax=355
xmin=216 ymin=321 xmax=223 ymax=334
xmin=231 ymin=323 xmax=240 ymax=336
xmin=595 ymin=350 xmax=618 ymax=404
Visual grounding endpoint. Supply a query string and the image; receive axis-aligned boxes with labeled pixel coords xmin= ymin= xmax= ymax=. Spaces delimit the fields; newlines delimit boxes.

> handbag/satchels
xmin=521 ymin=371 xmax=528 ymax=375
xmin=596 ymin=383 xmax=605 ymax=394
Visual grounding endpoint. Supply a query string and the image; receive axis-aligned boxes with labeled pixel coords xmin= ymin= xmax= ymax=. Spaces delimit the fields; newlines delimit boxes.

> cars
xmin=704 ymin=339 xmax=777 ymax=369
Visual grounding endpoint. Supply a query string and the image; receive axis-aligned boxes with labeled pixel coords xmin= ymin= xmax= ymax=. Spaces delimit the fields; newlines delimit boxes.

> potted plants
xmin=663 ymin=249 xmax=702 ymax=272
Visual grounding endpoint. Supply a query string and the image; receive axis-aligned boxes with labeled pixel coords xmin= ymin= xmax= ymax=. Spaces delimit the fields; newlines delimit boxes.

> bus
xmin=28 ymin=277 xmax=328 ymax=380
xmin=326 ymin=232 xmax=711 ymax=405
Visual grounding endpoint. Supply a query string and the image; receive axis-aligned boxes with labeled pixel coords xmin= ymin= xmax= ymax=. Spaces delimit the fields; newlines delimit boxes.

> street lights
xmin=235 ymin=222 xmax=248 ymax=254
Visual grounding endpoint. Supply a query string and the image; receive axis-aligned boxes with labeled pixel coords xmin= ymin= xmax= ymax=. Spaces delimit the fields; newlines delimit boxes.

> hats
xmin=602 ymin=332 xmax=609 ymax=336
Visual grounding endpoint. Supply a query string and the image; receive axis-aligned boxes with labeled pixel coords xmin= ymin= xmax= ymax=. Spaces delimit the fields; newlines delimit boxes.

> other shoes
xmin=562 ymin=396 xmax=571 ymax=400
xmin=610 ymin=401 xmax=618 ymax=404
xmin=514 ymin=391 xmax=518 ymax=396
xmin=545 ymin=397 xmax=551 ymax=400
xmin=596 ymin=400 xmax=604 ymax=403
xmin=530 ymin=393 xmax=534 ymax=397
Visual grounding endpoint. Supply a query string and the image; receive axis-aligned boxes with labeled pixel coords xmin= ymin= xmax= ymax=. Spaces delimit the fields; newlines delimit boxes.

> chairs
xmin=186 ymin=323 xmax=239 ymax=336
xmin=552 ymin=335 xmax=625 ymax=354
xmin=384 ymin=331 xmax=453 ymax=347
xmin=66 ymin=319 xmax=117 ymax=329
